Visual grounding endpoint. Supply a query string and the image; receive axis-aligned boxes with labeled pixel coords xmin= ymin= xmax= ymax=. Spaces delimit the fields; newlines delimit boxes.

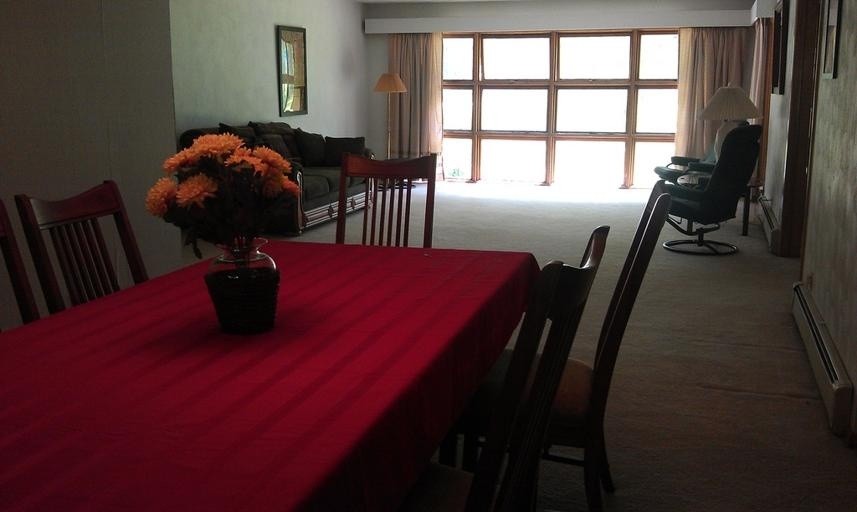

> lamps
xmin=698 ymin=77 xmax=764 ymax=169
xmin=370 ymin=71 xmax=408 ymax=189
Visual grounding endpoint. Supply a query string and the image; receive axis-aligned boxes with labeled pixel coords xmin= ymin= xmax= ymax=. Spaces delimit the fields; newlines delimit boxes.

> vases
xmin=199 ymin=235 xmax=279 ymax=338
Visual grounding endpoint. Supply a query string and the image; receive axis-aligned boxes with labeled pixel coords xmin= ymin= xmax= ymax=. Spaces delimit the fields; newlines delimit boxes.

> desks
xmin=681 ymin=169 xmax=764 ymax=239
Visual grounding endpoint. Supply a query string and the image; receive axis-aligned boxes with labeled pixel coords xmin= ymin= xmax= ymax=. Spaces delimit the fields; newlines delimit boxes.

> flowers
xmin=139 ymin=131 xmax=306 ymax=277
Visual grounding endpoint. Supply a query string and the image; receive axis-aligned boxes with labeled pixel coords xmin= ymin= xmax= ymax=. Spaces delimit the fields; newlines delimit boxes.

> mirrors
xmin=274 ymin=22 xmax=310 ymax=118
xmin=818 ymin=0 xmax=845 ymax=82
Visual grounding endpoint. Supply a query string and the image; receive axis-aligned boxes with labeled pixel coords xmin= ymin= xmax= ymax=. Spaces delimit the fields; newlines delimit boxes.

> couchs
xmin=174 ymin=120 xmax=378 ymax=238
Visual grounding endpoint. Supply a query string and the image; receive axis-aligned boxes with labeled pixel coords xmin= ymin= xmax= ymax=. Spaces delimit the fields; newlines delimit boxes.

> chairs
xmin=326 ymin=143 xmax=442 ymax=251
xmin=456 ymin=174 xmax=673 ymax=510
xmin=336 ymin=224 xmax=613 ymax=509
xmin=0 ymin=192 xmax=39 ymax=334
xmin=16 ymin=177 xmax=152 ymax=321
xmin=650 ymin=124 xmax=766 ymax=258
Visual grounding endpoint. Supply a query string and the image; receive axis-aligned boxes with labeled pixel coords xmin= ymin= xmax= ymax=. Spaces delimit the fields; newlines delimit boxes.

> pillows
xmin=213 ymin=118 xmax=368 ymax=171
